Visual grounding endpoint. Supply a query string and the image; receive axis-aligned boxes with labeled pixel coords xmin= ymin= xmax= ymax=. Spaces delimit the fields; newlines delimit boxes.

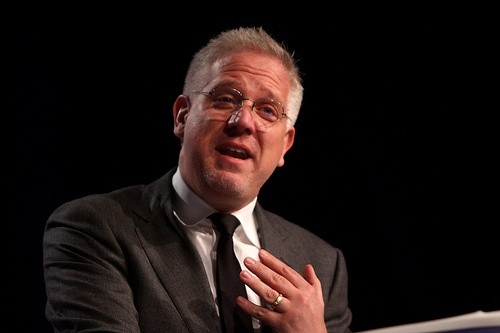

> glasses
xmin=194 ymin=86 xmax=289 ymax=124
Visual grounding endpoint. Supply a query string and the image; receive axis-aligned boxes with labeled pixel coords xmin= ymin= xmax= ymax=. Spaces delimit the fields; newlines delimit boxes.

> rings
xmin=268 ymin=294 xmax=283 ymax=308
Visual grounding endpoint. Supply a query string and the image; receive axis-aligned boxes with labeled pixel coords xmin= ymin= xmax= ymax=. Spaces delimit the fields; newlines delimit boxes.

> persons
xmin=42 ymin=27 xmax=351 ymax=333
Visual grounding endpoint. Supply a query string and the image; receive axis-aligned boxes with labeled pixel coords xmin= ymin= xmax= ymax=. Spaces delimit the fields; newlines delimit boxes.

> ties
xmin=205 ymin=211 xmax=254 ymax=333
